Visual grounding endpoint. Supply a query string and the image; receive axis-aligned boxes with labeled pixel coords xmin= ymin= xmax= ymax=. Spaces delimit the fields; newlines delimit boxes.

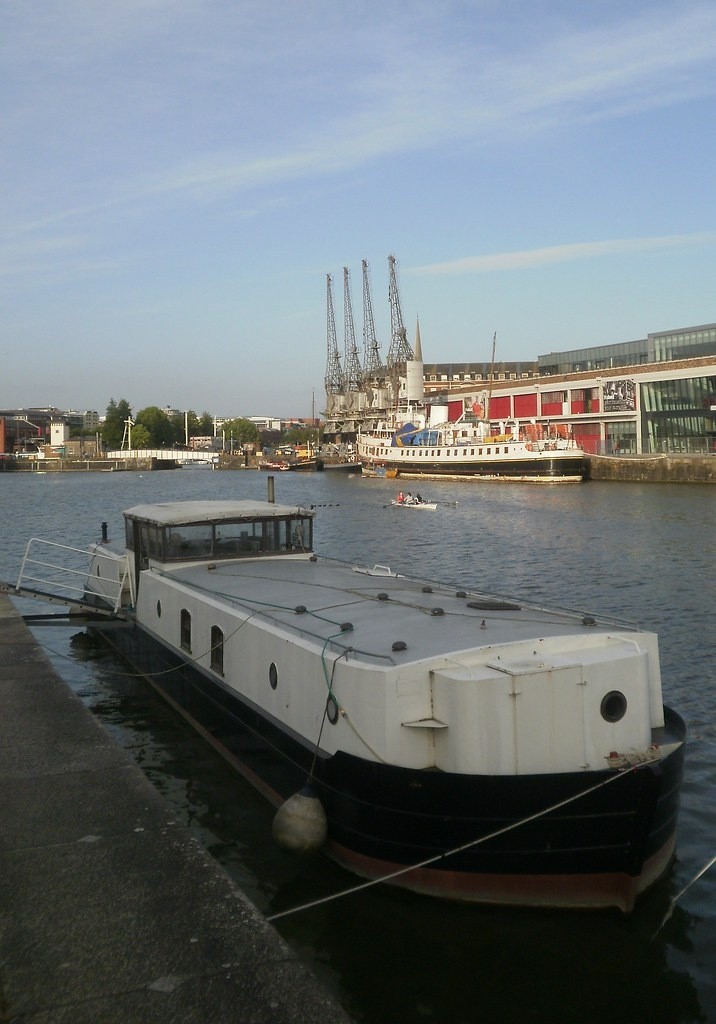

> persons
xmin=413 ymin=493 xmax=423 ymax=504
xmin=398 ymin=490 xmax=404 ymax=503
xmin=291 ymin=525 xmax=306 ymax=547
xmin=610 ymin=441 xmax=621 ymax=457
xmin=403 ymin=492 xmax=413 ymax=504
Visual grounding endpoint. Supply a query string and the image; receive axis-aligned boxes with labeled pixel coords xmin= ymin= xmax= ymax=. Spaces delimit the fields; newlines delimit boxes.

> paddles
xmin=427 ymin=500 xmax=462 ymax=509
xmin=382 ymin=501 xmax=400 ymax=509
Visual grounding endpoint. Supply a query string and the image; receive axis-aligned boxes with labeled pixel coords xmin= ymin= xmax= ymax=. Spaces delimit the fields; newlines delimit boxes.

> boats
xmin=258 ymin=459 xmax=322 ymax=472
xmin=355 ymin=417 xmax=586 ymax=483
xmin=390 ymin=500 xmax=438 ymax=510
xmin=81 ymin=474 xmax=688 ymax=913
